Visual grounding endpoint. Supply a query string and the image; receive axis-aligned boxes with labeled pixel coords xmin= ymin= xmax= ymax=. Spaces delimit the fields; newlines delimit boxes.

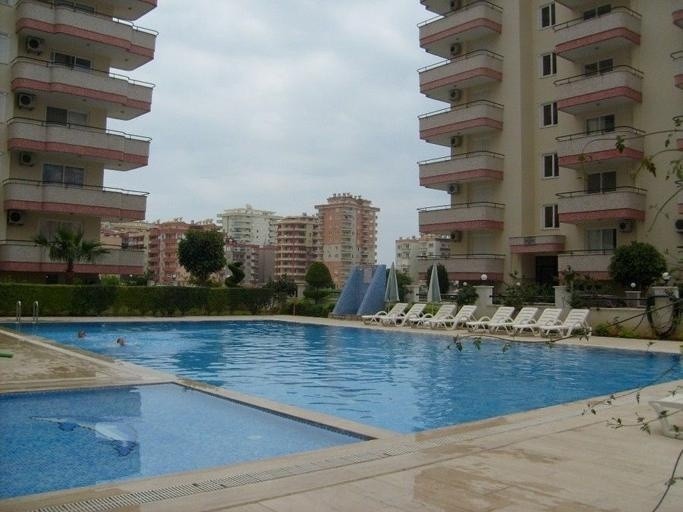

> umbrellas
xmin=383 ymin=261 xmax=400 ymax=312
xmin=424 ymin=261 xmax=441 ymax=315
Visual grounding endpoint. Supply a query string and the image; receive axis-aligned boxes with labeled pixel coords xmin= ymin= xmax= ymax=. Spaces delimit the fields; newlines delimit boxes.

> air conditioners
xmin=442 ymin=1 xmax=466 ymax=245
xmin=7 ymin=33 xmax=44 ymax=227
xmin=617 ymin=218 xmax=634 ymax=234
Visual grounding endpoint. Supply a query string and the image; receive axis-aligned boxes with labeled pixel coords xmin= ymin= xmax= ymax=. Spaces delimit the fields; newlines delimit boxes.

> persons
xmin=116 ymin=338 xmax=125 ymax=345
xmin=77 ymin=330 xmax=88 ymax=336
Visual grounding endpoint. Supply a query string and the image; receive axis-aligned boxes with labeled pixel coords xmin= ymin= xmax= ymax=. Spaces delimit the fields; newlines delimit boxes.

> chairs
xmin=357 ymin=301 xmax=593 ymax=342
